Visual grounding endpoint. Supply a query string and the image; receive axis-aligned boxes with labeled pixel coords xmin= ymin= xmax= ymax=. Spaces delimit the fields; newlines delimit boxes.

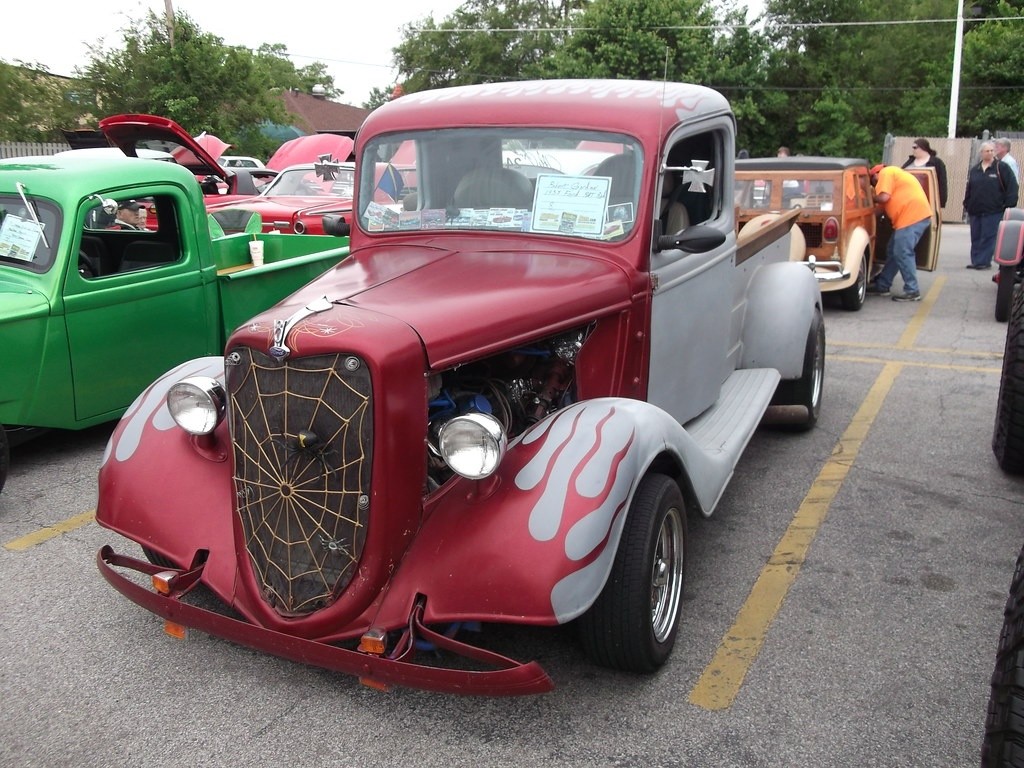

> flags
xmin=379 ymin=164 xmax=404 ymax=203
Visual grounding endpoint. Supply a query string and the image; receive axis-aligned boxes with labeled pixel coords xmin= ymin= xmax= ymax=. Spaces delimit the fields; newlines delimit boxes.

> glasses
xmin=912 ymin=146 xmax=919 ymax=150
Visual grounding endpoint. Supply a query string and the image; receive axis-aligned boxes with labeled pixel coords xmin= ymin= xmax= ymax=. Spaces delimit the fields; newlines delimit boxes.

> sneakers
xmin=865 ymin=287 xmax=890 ymax=296
xmin=893 ymin=291 xmax=921 ymax=302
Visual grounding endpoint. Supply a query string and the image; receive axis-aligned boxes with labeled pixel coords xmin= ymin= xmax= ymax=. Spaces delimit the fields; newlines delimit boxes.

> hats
xmin=993 ymin=137 xmax=1011 ymax=146
xmin=870 ymin=164 xmax=886 ymax=176
xmin=117 ymin=200 xmax=146 ymax=210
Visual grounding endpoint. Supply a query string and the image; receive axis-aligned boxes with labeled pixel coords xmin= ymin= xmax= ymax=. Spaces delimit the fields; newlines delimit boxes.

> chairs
xmin=764 ymin=187 xmax=805 ymax=213
xmin=117 ymin=241 xmax=177 ymax=277
xmin=592 ymin=153 xmax=640 ymax=211
xmin=450 ymin=165 xmax=533 ymax=210
xmin=81 ymin=235 xmax=113 ymax=273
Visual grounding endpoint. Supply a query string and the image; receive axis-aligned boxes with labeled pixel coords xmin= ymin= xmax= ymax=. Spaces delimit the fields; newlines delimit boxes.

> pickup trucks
xmin=0 ymin=147 xmax=352 ymax=492
xmin=94 ymin=79 xmax=826 ymax=696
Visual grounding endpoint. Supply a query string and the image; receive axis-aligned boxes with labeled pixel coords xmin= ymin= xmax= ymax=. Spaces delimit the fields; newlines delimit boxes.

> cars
xmin=200 ymin=154 xmax=418 ymax=239
xmin=980 ymin=165 xmax=1024 ymax=768
xmin=54 ymin=113 xmax=418 ymax=201
xmin=734 ymin=157 xmax=942 ymax=312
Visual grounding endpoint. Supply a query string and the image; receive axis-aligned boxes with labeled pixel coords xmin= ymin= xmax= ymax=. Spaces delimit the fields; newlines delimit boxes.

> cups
xmin=249 ymin=240 xmax=264 ymax=266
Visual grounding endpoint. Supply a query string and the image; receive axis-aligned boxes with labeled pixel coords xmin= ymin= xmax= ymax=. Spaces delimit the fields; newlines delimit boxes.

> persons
xmin=962 ymin=139 xmax=1019 ymax=270
xmin=776 ymin=146 xmax=790 ymax=158
xmin=105 ymin=200 xmax=152 ymax=232
xmin=900 ymin=139 xmax=947 ymax=213
xmin=995 ymin=135 xmax=1019 ymax=184
xmin=868 ymin=164 xmax=933 ymax=302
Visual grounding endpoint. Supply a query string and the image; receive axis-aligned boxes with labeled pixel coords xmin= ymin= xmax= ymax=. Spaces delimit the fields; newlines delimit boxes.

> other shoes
xmin=967 ymin=264 xmax=991 ymax=269
